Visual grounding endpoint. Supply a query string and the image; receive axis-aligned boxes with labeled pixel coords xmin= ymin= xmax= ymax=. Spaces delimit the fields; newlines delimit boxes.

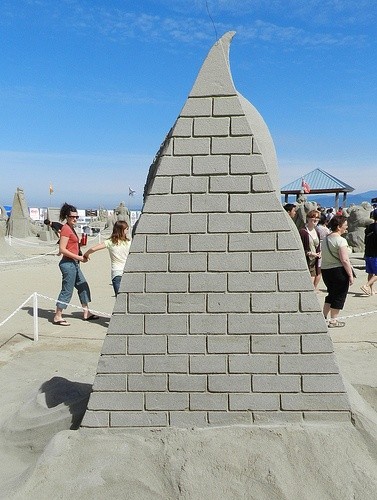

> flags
xmin=302 ymin=178 xmax=310 ymax=193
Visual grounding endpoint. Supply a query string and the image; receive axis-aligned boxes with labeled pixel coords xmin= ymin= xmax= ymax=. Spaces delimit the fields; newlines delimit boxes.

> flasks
xmin=82 ymin=233 xmax=87 ymax=245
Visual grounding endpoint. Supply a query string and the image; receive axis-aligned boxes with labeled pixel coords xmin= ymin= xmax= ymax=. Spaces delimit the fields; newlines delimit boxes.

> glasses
xmin=70 ymin=216 xmax=79 ymax=219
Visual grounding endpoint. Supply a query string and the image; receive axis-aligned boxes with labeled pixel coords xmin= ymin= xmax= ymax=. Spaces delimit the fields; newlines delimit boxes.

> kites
xmin=50 ymin=185 xmax=53 ymax=195
xmin=129 ymin=188 xmax=135 ymax=196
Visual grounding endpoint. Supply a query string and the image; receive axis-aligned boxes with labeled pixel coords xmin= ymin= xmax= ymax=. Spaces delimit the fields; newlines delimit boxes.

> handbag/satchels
xmin=351 ymin=265 xmax=357 ymax=279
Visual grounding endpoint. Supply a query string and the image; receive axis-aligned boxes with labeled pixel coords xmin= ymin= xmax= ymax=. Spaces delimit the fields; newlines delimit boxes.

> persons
xmin=320 ymin=215 xmax=354 ymax=328
xmin=361 ymin=209 xmax=377 ymax=296
xmin=44 ymin=219 xmax=64 ymax=256
xmin=83 ymin=220 xmax=133 ymax=298
xmin=372 ymin=204 xmax=377 ymax=210
xmin=284 ymin=204 xmax=351 ymax=293
xmin=53 ymin=203 xmax=100 ymax=326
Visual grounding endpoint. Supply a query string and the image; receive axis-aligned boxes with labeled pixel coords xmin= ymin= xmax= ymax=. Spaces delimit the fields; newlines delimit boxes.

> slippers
xmin=52 ymin=321 xmax=70 ymax=326
xmin=83 ymin=315 xmax=99 ymax=320
xmin=360 ymin=287 xmax=370 ymax=295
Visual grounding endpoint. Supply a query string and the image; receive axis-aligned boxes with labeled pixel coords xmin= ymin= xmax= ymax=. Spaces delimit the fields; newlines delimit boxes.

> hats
xmin=371 ymin=198 xmax=377 ymax=205
xmin=373 ymin=209 xmax=377 ymax=217
xmin=307 ymin=210 xmax=321 ymax=220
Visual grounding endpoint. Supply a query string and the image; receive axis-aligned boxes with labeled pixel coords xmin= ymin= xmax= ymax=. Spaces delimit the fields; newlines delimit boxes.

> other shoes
xmin=328 ymin=319 xmax=345 ymax=327
